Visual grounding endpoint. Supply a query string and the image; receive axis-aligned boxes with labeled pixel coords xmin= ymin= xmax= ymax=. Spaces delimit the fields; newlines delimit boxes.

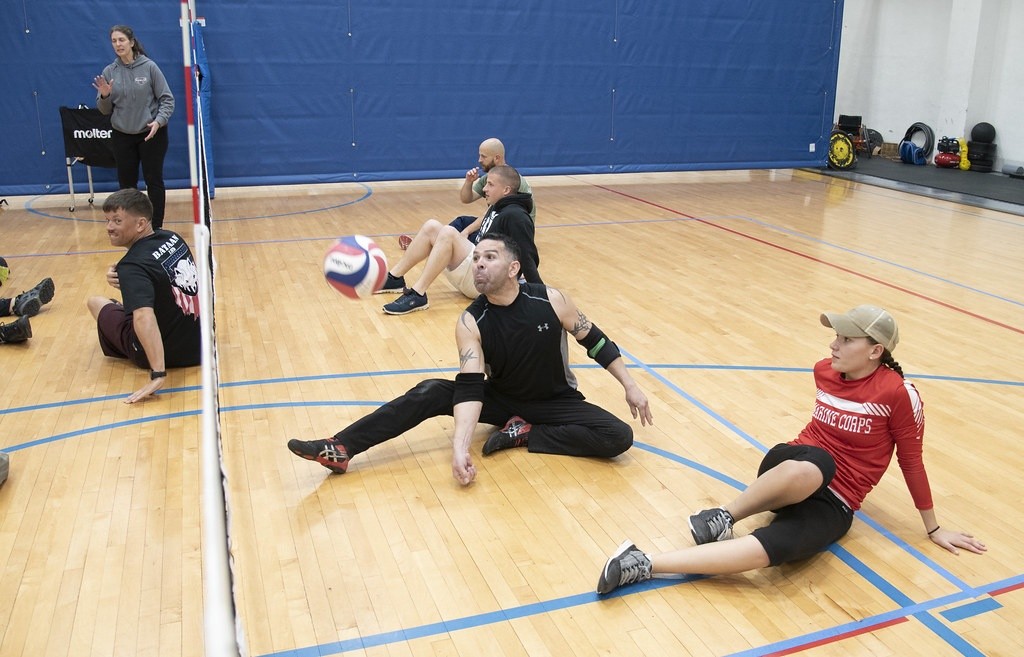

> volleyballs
xmin=323 ymin=235 xmax=388 ymax=300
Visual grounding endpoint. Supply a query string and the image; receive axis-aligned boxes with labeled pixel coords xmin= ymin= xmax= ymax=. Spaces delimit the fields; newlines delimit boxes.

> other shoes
xmin=399 ymin=235 xmax=413 ymax=250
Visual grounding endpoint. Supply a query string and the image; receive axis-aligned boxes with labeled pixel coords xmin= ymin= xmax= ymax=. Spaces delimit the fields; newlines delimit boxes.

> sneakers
xmin=382 ymin=288 xmax=428 ymax=315
xmin=13 ymin=278 xmax=54 ymax=318
xmin=598 ymin=538 xmax=651 ymax=595
xmin=481 ymin=414 xmax=533 ymax=454
xmin=372 ymin=273 xmax=407 ymax=295
xmin=689 ymin=504 xmax=735 ymax=547
xmin=288 ymin=435 xmax=351 ymax=474
xmin=0 ymin=315 xmax=32 ymax=344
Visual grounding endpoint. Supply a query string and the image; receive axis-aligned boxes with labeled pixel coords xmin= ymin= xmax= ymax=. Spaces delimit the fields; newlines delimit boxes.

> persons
xmin=0 ymin=277 xmax=55 ymax=343
xmin=91 ymin=25 xmax=176 ymax=229
xmin=398 ymin=138 xmax=536 ymax=253
xmin=288 ymin=233 xmax=653 ymax=485
xmin=596 ymin=305 xmax=988 ymax=594
xmin=374 ymin=165 xmax=540 ymax=313
xmin=87 ymin=189 xmax=202 ymax=404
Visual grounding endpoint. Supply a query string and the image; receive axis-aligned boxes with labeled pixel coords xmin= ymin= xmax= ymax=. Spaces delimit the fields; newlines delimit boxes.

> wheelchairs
xmin=832 ymin=114 xmax=873 ymax=158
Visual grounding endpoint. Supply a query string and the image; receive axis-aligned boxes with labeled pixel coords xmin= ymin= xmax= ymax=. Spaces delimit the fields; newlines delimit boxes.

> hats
xmin=819 ymin=305 xmax=899 ymax=352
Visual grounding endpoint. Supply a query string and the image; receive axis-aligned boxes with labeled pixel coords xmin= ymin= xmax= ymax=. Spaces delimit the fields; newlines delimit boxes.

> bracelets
xmin=151 ymin=371 xmax=167 ymax=380
xmin=928 ymin=526 xmax=940 ymax=534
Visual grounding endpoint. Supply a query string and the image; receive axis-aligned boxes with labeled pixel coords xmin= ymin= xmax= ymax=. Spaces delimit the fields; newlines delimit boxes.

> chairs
xmin=59 ymin=104 xmax=118 ymax=213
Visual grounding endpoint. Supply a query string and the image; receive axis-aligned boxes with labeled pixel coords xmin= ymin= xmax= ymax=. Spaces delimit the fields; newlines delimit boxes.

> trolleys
xmin=59 ymin=102 xmax=119 ymax=212
